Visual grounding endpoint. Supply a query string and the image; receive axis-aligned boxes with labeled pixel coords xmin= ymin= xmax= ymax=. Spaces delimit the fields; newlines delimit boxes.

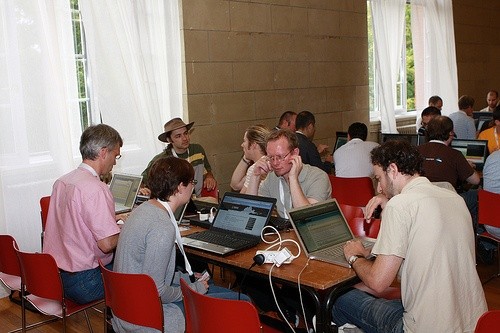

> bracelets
xmin=242 ymin=158 xmax=251 ymax=166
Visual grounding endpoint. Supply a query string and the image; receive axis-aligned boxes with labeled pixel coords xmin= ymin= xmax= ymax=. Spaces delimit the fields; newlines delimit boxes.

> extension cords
xmin=255 ymin=250 xmax=291 ymax=264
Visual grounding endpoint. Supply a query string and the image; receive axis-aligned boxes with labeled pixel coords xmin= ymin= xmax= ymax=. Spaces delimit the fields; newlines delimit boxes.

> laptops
xmin=382 ymin=133 xmax=418 ymax=146
xmin=286 ymin=199 xmax=378 ymax=268
xmin=109 ymin=173 xmax=143 ymax=212
xmin=332 ymin=135 xmax=347 ymax=155
xmin=450 ymin=138 xmax=488 ymax=171
xmin=174 ymin=191 xmax=277 ymax=256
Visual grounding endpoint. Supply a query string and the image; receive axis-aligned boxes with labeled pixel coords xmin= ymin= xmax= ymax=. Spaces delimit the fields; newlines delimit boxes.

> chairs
xmin=0 ymin=174 xmax=500 ymax=333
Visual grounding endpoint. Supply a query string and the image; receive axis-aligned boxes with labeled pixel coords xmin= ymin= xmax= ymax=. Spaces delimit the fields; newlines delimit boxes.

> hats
xmin=158 ymin=118 xmax=194 ymax=143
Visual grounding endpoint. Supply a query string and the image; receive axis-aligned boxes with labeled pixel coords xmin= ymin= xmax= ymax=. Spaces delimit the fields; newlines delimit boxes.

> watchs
xmin=348 ymin=255 xmax=366 ymax=269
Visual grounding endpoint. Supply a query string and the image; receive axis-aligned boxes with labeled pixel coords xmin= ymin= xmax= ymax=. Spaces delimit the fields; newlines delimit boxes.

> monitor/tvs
xmin=471 ymin=111 xmax=495 ymax=133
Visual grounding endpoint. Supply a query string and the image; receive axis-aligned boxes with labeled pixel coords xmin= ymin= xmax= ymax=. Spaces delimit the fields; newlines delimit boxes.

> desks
xmin=111 ymin=206 xmax=377 ymax=333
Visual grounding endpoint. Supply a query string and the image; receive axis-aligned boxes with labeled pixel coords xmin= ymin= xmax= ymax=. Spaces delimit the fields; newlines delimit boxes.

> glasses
xmin=421 ymin=121 xmax=429 ymax=127
xmin=178 ymin=180 xmax=198 ymax=186
xmin=267 ymin=146 xmax=297 ymax=163
xmin=101 ymin=147 xmax=121 ymax=159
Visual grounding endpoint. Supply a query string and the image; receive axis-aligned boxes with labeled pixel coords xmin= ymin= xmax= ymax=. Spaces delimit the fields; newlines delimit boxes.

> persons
xmin=270 ymin=112 xmax=299 ymax=140
xmin=318 ymin=141 xmax=489 ymax=333
xmin=418 ymin=106 xmax=442 ymax=135
xmin=415 ymin=116 xmax=486 ymax=261
xmin=43 ymin=124 xmax=195 ymax=333
xmin=479 ymin=90 xmax=500 ymax=112
xmin=135 ymin=118 xmax=217 ymax=283
xmin=482 ymin=149 xmax=500 ymax=261
xmin=290 ymin=111 xmax=336 ymax=174
xmin=478 ymin=104 xmax=500 ymax=154
xmin=240 ymin=129 xmax=333 ymax=322
xmin=416 ymin=96 xmax=443 ymax=134
xmin=333 ymin=122 xmax=382 ymax=178
xmin=229 ymin=125 xmax=275 ymax=191
xmin=112 ymin=156 xmax=252 ymax=333
xmin=447 ymin=95 xmax=478 ymax=139
